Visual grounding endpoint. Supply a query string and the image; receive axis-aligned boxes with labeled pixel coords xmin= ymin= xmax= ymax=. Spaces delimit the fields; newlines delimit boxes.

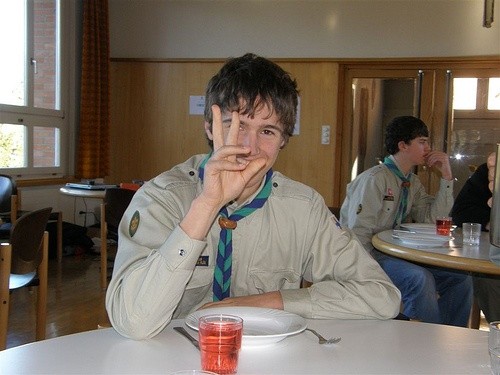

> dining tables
xmin=0 ymin=319 xmax=496 ymax=375
xmin=371 ymin=229 xmax=500 ymax=330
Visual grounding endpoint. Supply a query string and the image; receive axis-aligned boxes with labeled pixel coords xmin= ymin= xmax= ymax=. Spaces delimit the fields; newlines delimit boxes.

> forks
xmin=307 ymin=328 xmax=341 ymax=345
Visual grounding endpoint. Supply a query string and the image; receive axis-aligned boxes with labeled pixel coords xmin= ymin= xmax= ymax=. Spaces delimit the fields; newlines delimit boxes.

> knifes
xmin=174 ymin=327 xmax=201 ymax=351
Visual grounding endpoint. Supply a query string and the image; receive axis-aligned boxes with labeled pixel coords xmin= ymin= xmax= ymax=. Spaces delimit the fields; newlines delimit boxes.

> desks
xmin=60 ymin=187 xmax=107 ymax=291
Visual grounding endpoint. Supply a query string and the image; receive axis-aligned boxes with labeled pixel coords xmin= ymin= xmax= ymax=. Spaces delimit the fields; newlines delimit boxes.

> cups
xmin=489 ymin=142 xmax=500 ymax=248
xmin=435 ymin=215 xmax=453 ymax=236
xmin=463 ymin=224 xmax=480 ymax=247
xmin=489 ymin=320 xmax=500 ymax=375
xmin=199 ymin=314 xmax=243 ymax=374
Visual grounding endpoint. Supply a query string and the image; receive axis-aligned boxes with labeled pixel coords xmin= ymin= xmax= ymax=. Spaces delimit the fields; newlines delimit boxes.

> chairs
xmin=0 ymin=175 xmax=52 ymax=350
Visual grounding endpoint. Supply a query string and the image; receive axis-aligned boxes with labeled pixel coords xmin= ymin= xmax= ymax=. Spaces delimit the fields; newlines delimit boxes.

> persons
xmin=339 ymin=115 xmax=474 ymax=328
xmin=105 ymin=51 xmax=401 ymax=341
xmin=447 ymin=151 xmax=500 ymax=325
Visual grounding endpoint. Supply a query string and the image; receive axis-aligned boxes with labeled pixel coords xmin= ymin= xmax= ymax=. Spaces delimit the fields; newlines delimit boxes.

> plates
xmin=186 ymin=305 xmax=307 ymax=344
xmin=392 ymin=222 xmax=456 ymax=247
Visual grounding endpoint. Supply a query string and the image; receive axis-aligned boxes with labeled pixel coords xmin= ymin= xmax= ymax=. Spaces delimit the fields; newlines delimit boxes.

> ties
xmin=198 ymin=151 xmax=272 ymax=302
xmin=384 ymin=156 xmax=419 ymax=230
